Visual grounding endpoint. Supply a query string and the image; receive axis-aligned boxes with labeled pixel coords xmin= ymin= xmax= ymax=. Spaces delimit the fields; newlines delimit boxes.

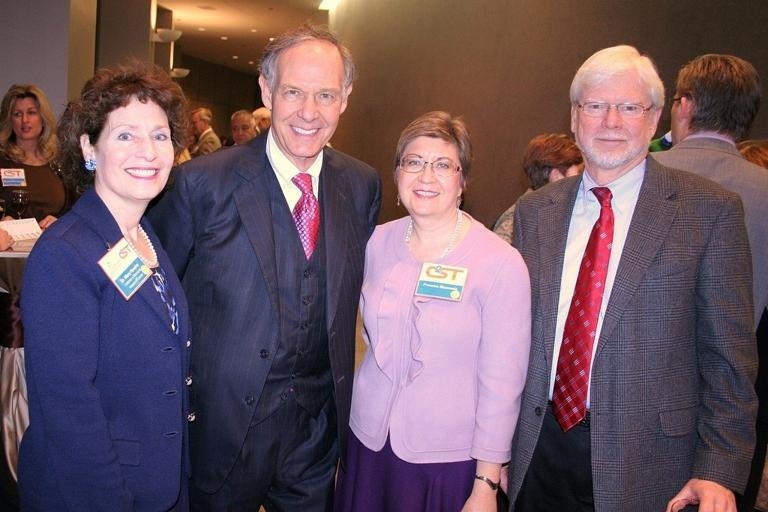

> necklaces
xmin=114 ymin=222 xmax=159 ymax=267
xmin=404 ymin=209 xmax=465 ymax=266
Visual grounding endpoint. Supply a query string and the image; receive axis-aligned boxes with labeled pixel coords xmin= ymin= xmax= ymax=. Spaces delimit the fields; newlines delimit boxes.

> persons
xmin=506 ymin=43 xmax=756 ymax=512
xmin=349 ymin=109 xmax=536 ymax=512
xmin=145 ymin=22 xmax=382 ymax=512
xmin=0 ymin=228 xmax=14 ymax=252
xmin=251 ymin=106 xmax=272 ymax=134
xmin=492 ymin=129 xmax=585 ymax=255
xmin=648 ymin=54 xmax=768 ymax=337
xmin=189 ymin=102 xmax=223 ymax=160
xmin=229 ymin=108 xmax=256 ymax=148
xmin=16 ymin=53 xmax=191 ymax=511
xmin=0 ymin=82 xmax=78 ymax=486
xmin=737 ymin=138 xmax=768 ymax=172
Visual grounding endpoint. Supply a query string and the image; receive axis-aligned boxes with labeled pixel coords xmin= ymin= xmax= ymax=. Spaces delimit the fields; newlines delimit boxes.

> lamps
xmin=171 ymin=68 xmax=190 ymax=78
xmin=156 ymin=28 xmax=182 ymax=41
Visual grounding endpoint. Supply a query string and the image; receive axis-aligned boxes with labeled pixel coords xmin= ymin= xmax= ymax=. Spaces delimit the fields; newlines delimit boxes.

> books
xmin=0 ymin=216 xmax=45 ymax=254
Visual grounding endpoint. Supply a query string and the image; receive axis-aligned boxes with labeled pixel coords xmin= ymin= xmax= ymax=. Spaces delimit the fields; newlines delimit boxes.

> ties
xmin=551 ymin=187 xmax=613 ymax=433
xmin=289 ymin=173 xmax=321 ymax=261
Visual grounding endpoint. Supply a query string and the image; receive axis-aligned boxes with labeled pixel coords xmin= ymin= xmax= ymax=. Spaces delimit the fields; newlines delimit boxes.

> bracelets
xmin=474 ymin=472 xmax=502 ymax=493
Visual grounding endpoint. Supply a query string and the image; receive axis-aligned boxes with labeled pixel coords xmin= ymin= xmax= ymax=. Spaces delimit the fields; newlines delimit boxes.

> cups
xmin=671 ymin=499 xmax=699 ymax=512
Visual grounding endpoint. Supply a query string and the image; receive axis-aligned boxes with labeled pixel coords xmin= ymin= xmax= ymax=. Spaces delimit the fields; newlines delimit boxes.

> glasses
xmin=395 ymin=153 xmax=464 ymax=178
xmin=275 ymin=86 xmax=344 ymax=107
xmin=575 ymin=102 xmax=654 ymax=119
xmin=667 ymin=97 xmax=682 ymax=108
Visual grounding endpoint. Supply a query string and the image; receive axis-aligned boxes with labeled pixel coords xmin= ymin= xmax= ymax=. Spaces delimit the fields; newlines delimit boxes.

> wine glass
xmin=9 ymin=190 xmax=30 ymax=219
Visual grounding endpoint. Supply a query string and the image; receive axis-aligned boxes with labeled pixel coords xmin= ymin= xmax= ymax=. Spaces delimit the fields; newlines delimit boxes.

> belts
xmin=546 ymin=401 xmax=591 ymax=429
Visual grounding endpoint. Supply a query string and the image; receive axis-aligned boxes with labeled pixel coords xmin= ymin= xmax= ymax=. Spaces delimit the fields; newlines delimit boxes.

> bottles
xmin=649 ymin=129 xmax=675 ymax=152
